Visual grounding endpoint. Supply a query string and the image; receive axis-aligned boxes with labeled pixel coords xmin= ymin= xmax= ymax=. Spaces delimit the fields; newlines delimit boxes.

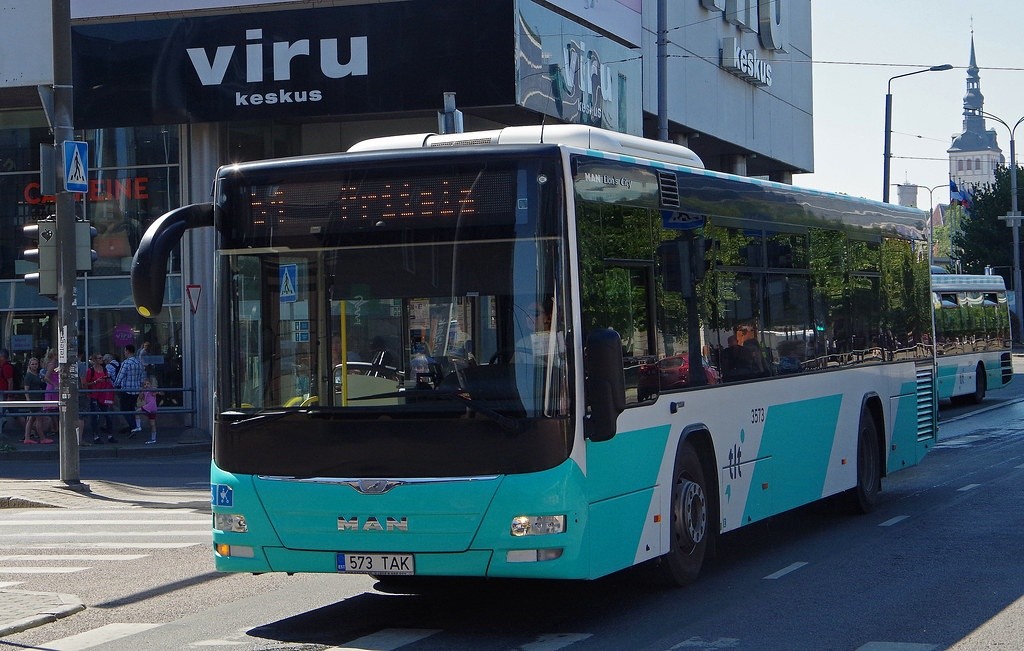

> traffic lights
xmin=74 ymin=220 xmax=97 ymax=273
xmin=23 ymin=221 xmax=58 ymax=297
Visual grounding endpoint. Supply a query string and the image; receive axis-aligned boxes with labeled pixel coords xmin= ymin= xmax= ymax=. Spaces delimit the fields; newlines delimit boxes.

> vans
xmin=755 ymin=328 xmax=824 ymax=370
xmin=516 ymin=330 xmax=597 ymax=377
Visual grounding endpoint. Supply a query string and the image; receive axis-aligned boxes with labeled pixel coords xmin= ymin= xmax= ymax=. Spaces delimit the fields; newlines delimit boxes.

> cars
xmin=639 ymin=354 xmax=720 ymax=388
xmin=624 ymin=354 xmax=655 ymax=389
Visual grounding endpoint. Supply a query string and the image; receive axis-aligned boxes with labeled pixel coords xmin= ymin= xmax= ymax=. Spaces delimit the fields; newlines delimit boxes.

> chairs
xmin=571 ymin=338 xmax=868 ymax=441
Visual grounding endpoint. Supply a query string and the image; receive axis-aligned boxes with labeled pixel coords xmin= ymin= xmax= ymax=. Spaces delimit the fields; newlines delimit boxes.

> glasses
xmin=95 ymin=357 xmax=103 ymax=360
xmin=31 ymin=363 xmax=38 ymax=365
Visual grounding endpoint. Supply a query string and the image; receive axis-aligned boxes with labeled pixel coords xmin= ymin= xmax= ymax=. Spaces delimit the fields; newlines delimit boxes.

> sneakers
xmin=145 ymin=439 xmax=156 ymax=445
xmin=78 ymin=441 xmax=91 ymax=446
xmin=127 ymin=431 xmax=138 ymax=439
xmin=93 ymin=438 xmax=104 ymax=444
xmin=40 ymin=439 xmax=54 ymax=444
xmin=24 ymin=440 xmax=38 ymax=444
xmin=107 ymin=436 xmax=118 ymax=443
xmin=131 ymin=427 xmax=143 ymax=432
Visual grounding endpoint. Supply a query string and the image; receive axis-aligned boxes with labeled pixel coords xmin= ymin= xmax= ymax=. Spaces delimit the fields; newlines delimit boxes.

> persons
xmin=137 ymin=342 xmax=155 ymax=380
xmin=331 ymin=317 xmax=470 ymax=383
xmin=0 ymin=345 xmax=158 ymax=446
xmin=719 ymin=333 xmax=774 ymax=382
xmin=508 ymin=294 xmax=569 ymax=411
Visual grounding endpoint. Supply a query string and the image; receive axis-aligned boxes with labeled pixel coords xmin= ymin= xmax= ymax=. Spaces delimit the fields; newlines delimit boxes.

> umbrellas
xmin=974 ymin=300 xmax=997 ymax=344
xmin=934 ymin=300 xmax=958 ymax=343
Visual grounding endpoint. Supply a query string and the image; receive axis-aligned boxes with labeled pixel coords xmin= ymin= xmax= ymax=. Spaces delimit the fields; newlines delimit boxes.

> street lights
xmin=883 ymin=65 xmax=953 ymax=203
xmin=962 ymin=107 xmax=1024 ymax=343
xmin=890 ymin=183 xmax=959 ymax=265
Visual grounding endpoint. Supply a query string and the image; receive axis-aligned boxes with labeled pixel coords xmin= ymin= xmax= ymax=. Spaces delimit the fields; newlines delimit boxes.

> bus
xmin=929 ymin=266 xmax=1013 ymax=406
xmin=131 ymin=123 xmax=937 ymax=583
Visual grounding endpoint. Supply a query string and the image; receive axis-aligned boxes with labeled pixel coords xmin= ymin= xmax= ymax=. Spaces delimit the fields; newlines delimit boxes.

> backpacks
xmin=107 ymin=362 xmax=122 ymax=389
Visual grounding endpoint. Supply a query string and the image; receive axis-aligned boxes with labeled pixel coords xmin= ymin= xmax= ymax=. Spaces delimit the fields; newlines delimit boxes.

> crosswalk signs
xmin=661 ymin=210 xmax=707 ymax=230
xmin=279 ymin=265 xmax=298 ymax=302
xmin=63 ymin=142 xmax=91 ymax=195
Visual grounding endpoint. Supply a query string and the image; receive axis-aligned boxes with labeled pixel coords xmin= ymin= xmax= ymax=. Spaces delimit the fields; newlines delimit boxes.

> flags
xmin=957 ymin=186 xmax=974 ymax=215
xmin=950 ymin=179 xmax=963 ymax=201
